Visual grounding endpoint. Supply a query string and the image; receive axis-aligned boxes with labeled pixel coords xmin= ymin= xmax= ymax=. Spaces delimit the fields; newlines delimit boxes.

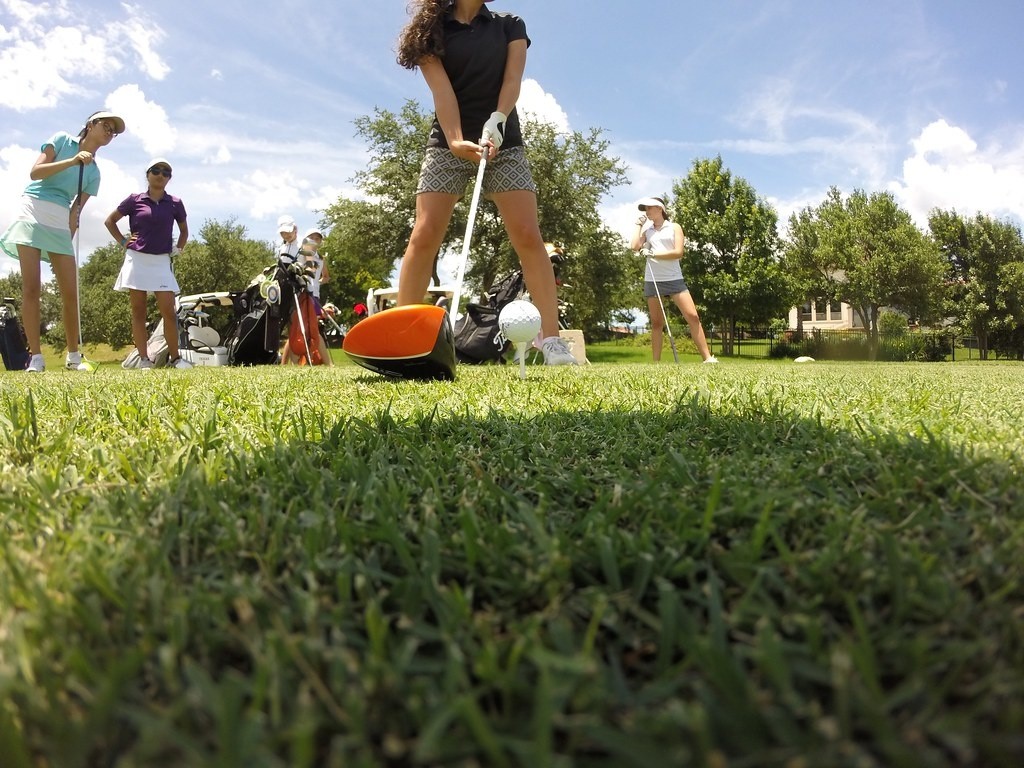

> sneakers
xmin=26 ymin=354 xmax=44 ymax=373
xmin=65 ymin=354 xmax=92 ymax=371
xmin=169 ymin=357 xmax=193 ymax=369
xmin=140 ymin=357 xmax=153 ymax=370
xmin=532 ymin=331 xmax=578 ymax=365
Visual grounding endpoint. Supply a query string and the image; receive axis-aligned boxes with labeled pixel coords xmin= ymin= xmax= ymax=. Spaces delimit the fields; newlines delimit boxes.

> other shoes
xmin=703 ymin=354 xmax=720 ymax=364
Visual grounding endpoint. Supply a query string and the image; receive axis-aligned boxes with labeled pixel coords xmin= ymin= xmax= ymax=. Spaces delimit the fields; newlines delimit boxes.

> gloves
xmin=170 ymin=245 xmax=182 ymax=258
xmin=635 ymin=214 xmax=647 ymax=226
xmin=640 ymin=248 xmax=655 ymax=258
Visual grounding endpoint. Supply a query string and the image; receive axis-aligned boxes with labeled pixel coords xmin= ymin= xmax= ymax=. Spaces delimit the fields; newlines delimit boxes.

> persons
xmin=0 ymin=111 xmax=126 ymax=373
xmin=277 ymin=215 xmax=332 ymax=367
xmin=104 ymin=159 xmax=194 ymax=368
xmin=632 ymin=198 xmax=719 ymax=362
xmin=397 ymin=0 xmax=577 ymax=364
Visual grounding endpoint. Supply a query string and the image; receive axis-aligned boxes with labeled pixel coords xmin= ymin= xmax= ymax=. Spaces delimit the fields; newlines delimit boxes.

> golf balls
xmin=498 ymin=299 xmax=542 ymax=341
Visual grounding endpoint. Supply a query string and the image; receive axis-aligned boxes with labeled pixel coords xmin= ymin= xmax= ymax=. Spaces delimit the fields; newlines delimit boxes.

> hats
xmin=638 ymin=199 xmax=666 ymax=211
xmin=146 ymin=157 xmax=173 ymax=175
xmin=305 ymin=230 xmax=323 ymax=239
xmin=86 ymin=112 xmax=125 ymax=134
xmin=279 ymin=219 xmax=297 ymax=233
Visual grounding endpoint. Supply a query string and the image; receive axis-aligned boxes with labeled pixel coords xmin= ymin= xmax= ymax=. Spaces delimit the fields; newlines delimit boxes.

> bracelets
xmin=121 ymin=239 xmax=127 ymax=246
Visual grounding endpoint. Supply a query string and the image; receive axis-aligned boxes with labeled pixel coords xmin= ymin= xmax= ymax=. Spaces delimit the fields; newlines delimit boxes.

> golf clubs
xmin=342 ymin=145 xmax=489 ymax=382
xmin=646 ymin=256 xmax=679 ymax=363
xmin=287 ymin=238 xmax=319 ymax=287
xmin=75 ymin=160 xmax=100 ymax=372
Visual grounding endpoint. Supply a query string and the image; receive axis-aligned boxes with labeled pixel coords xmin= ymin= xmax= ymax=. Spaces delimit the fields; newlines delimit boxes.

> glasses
xmin=149 ymin=168 xmax=170 ymax=177
xmin=99 ymin=121 xmax=117 ymax=139
